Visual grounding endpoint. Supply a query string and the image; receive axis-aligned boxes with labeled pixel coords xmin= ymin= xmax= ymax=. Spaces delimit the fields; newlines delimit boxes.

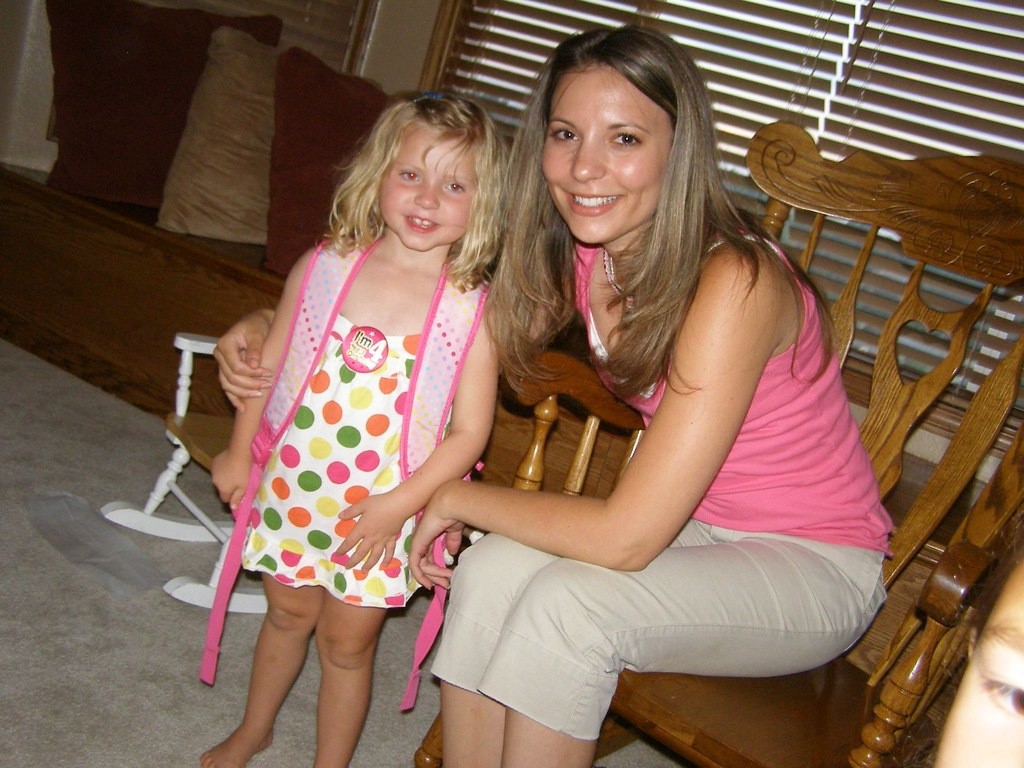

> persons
xmin=931 ymin=518 xmax=1024 ymax=768
xmin=199 ymin=90 xmax=507 ymax=768
xmin=408 ymin=25 xmax=893 ymax=768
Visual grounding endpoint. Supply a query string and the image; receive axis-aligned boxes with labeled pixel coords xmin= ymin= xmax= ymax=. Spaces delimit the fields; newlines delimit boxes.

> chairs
xmin=409 ymin=119 xmax=1024 ymax=768
xmin=98 ymin=330 xmax=272 ymax=615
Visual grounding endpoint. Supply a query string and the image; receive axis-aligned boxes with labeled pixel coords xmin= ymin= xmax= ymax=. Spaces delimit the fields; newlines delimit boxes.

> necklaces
xmin=603 ymin=248 xmax=634 ymax=309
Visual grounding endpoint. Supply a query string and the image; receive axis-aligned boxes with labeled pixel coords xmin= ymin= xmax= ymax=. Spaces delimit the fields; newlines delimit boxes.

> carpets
xmin=0 ymin=332 xmax=691 ymax=768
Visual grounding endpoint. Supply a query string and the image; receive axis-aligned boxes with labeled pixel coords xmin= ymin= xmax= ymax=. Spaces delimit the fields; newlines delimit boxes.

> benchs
xmin=0 ymin=162 xmax=985 ymax=614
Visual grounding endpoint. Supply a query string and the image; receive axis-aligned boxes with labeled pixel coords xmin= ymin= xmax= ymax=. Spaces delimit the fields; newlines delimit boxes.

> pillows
xmin=263 ymin=46 xmax=388 ymax=277
xmin=151 ymin=26 xmax=276 ymax=246
xmin=45 ymin=0 xmax=284 ymax=206
